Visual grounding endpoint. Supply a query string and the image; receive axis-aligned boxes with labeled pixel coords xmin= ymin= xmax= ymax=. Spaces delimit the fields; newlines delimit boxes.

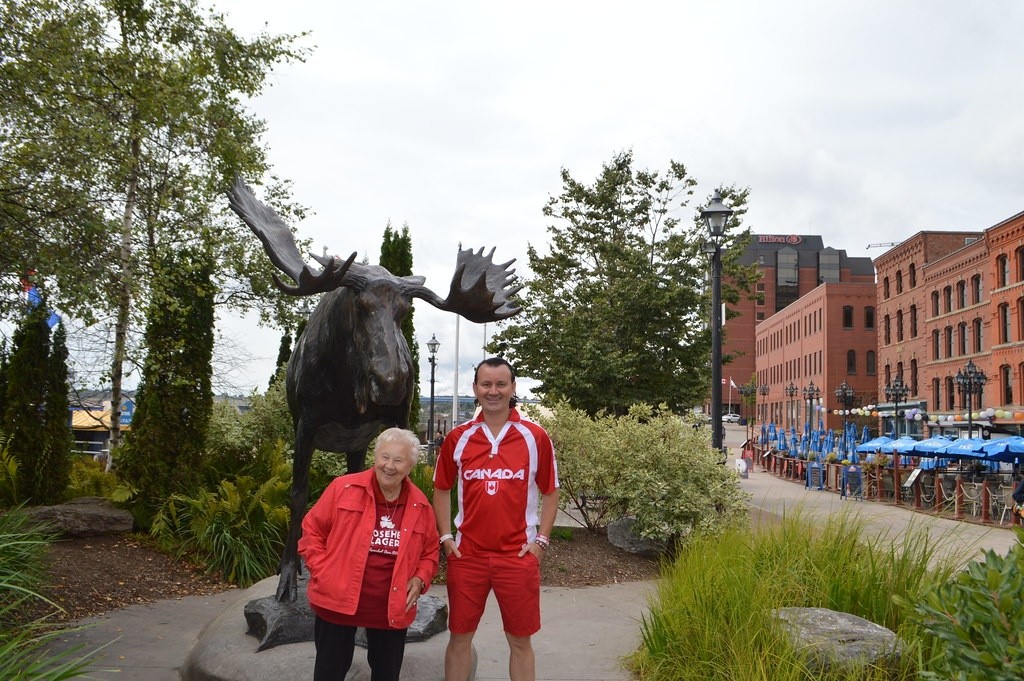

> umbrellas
xmin=837 ymin=421 xmax=855 ymax=466
xmin=758 ymin=418 xmax=834 ymax=457
xmin=855 ymin=425 xmax=1024 ymax=473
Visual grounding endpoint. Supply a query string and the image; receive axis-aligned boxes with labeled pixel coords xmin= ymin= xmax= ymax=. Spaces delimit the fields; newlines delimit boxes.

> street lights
xmin=759 ymin=384 xmax=770 ymax=424
xmin=784 ymin=381 xmax=799 ymax=427
xmin=882 ymin=375 xmax=911 ymax=442
xmin=699 ymin=192 xmax=731 ymax=453
xmin=834 ymin=380 xmax=855 ymax=448
xmin=802 ymin=380 xmax=821 ymax=442
xmin=427 ymin=333 xmax=440 ymax=465
xmin=738 ymin=384 xmax=758 ymax=443
xmin=955 ymin=358 xmax=989 ymax=438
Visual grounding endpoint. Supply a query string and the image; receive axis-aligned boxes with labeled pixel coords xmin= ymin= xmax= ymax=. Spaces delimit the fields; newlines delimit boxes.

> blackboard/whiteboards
xmin=805 ymin=462 xmax=823 ymax=487
xmin=840 ymin=466 xmax=863 ymax=498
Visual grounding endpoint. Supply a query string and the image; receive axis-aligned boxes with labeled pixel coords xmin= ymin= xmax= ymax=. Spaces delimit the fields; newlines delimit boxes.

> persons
xmin=297 ymin=427 xmax=440 ymax=681
xmin=1012 ymin=478 xmax=1024 ymax=519
xmin=432 ymin=357 xmax=560 ymax=681
xmin=434 ymin=431 xmax=444 ymax=457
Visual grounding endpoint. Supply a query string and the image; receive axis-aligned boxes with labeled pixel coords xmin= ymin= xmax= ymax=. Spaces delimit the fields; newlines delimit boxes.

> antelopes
xmin=223 ymin=170 xmax=525 ymax=605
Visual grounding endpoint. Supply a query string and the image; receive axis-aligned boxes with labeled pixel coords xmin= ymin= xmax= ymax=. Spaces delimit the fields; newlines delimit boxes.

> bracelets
xmin=438 ymin=533 xmax=455 ymax=543
xmin=421 ymin=581 xmax=425 ymax=589
xmin=534 ymin=532 xmax=551 ymax=551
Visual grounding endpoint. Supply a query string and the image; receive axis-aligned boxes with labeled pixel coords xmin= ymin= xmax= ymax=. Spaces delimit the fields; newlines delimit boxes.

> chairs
xmin=786 ymin=460 xmax=1017 ymax=525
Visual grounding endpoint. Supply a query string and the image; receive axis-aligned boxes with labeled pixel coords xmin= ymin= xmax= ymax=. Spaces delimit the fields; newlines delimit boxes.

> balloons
xmin=878 ymin=410 xmax=928 ymax=421
xmin=851 ymin=406 xmax=878 ymax=416
xmin=929 ymin=408 xmax=1024 ymax=421
xmin=814 ymin=403 xmax=850 ymax=416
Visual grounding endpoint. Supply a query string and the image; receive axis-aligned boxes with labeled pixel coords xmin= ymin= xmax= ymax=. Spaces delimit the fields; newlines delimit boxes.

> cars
xmin=700 ymin=415 xmax=711 ymax=424
xmin=720 ymin=414 xmax=739 ymax=423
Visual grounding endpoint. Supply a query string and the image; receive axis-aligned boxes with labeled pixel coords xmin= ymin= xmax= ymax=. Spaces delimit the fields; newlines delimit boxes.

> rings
xmin=412 ymin=603 xmax=417 ymax=606
